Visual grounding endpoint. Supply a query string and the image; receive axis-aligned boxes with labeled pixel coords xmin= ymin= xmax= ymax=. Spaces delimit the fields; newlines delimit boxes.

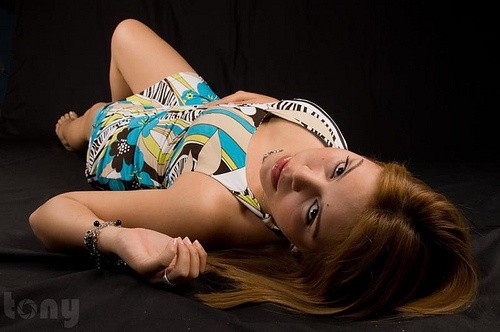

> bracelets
xmin=84 ymin=220 xmax=127 ymax=271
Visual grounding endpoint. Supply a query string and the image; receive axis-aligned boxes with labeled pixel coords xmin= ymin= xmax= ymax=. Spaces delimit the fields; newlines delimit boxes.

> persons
xmin=28 ymin=18 xmax=481 ymax=323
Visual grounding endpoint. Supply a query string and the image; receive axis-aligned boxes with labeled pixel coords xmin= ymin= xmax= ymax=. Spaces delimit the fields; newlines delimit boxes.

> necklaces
xmin=263 ymin=149 xmax=285 ymax=161
